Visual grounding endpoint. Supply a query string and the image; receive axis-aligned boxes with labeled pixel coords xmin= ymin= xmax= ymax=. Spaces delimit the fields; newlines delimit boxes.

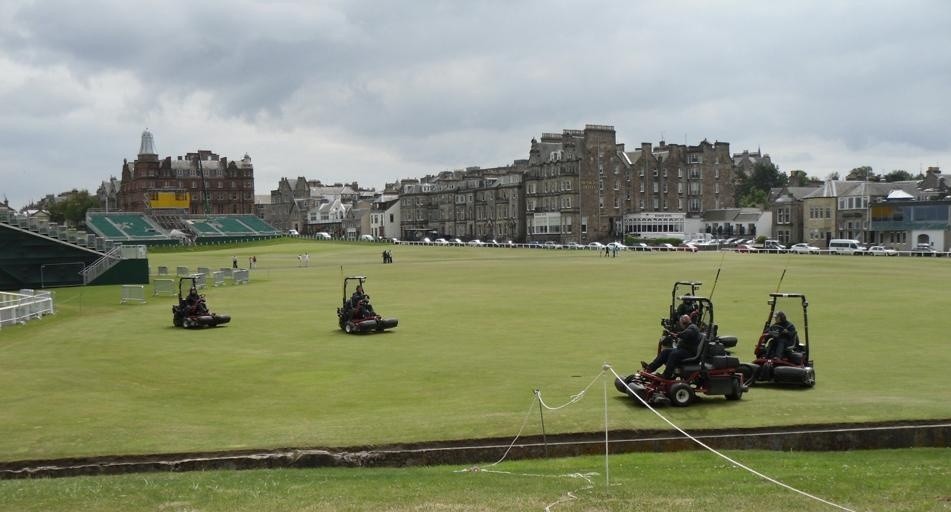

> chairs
xmin=680 ymin=333 xmax=707 ymax=362
xmin=788 ymin=329 xmax=799 ymax=350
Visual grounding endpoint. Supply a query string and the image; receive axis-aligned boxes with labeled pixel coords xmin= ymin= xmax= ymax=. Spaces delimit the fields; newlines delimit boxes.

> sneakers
xmin=640 ymin=359 xmax=653 ymax=373
xmin=655 ymin=371 xmax=673 ymax=380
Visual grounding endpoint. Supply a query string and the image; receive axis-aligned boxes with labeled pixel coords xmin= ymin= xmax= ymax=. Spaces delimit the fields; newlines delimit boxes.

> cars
xmin=288 ymin=229 xmax=300 ymax=235
xmin=420 ymin=237 xmax=517 ymax=248
xmin=316 ymin=232 xmax=331 ymax=239
xmin=362 ymin=235 xmax=401 ymax=244
xmin=829 ymin=238 xmax=940 ymax=256
xmin=528 ymin=237 xmax=820 ymax=254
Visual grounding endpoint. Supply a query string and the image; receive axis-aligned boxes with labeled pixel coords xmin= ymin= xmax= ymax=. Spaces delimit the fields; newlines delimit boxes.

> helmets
xmin=190 ymin=287 xmax=198 ymax=296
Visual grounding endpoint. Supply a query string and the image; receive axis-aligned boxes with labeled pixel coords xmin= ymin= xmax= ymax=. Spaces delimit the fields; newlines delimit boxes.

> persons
xmin=249 ymin=256 xmax=252 ymax=269
xmin=297 ymin=254 xmax=302 ymax=265
xmin=765 ymin=311 xmax=796 ymax=361
xmin=184 ymin=287 xmax=207 ymax=309
xmin=677 ymin=292 xmax=708 ymax=331
xmin=640 ymin=314 xmax=700 ymax=380
xmin=383 ymin=249 xmax=393 ymax=263
xmin=304 ymin=253 xmax=309 ymax=265
xmin=232 ymin=255 xmax=237 ymax=268
xmin=352 ymin=285 xmax=374 ymax=317
xmin=252 ymin=255 xmax=256 ymax=269
xmin=599 ymin=245 xmax=619 ymax=258
